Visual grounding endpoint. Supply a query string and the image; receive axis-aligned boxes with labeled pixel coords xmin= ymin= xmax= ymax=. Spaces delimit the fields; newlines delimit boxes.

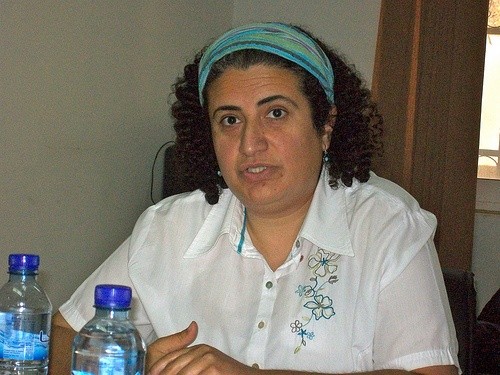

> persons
xmin=48 ymin=19 xmax=464 ymax=375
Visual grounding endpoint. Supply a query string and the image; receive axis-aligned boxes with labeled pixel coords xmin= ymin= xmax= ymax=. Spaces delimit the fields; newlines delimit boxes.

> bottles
xmin=0 ymin=253 xmax=53 ymax=375
xmin=69 ymin=284 xmax=147 ymax=375
